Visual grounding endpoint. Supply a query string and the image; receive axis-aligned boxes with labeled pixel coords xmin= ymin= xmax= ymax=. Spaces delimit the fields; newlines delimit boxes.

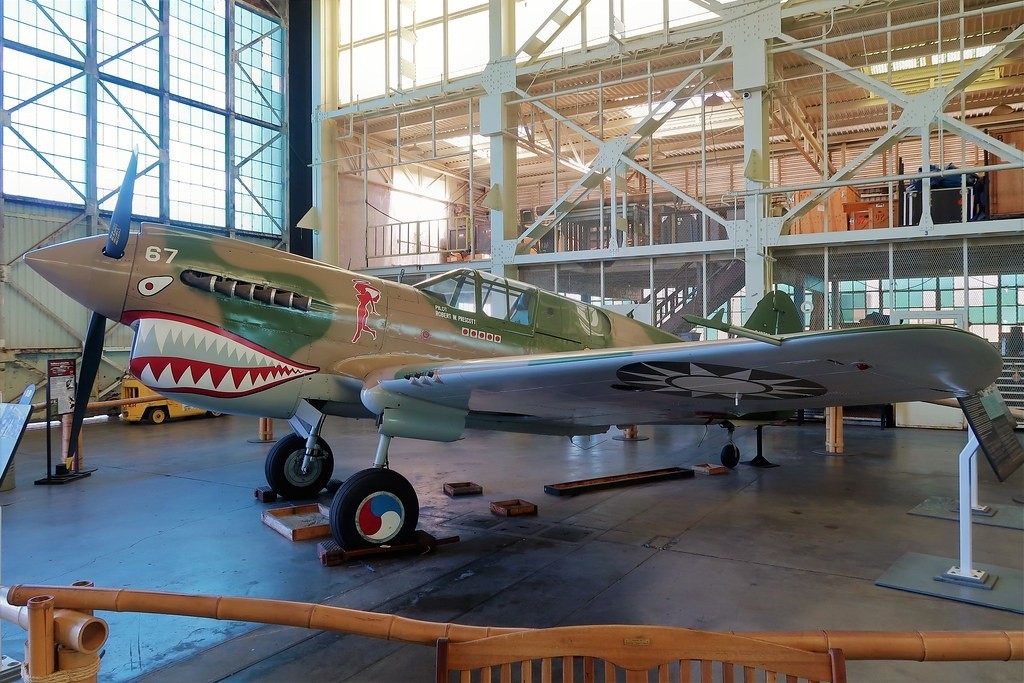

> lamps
xmin=590 ymin=112 xmax=608 ymax=126
xmin=705 ymin=83 xmax=725 ymax=106
xmin=407 ymin=133 xmax=424 ymax=154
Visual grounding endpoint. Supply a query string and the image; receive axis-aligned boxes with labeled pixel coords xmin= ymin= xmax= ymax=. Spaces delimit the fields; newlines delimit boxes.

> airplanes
xmin=23 ymin=146 xmax=1005 ymax=556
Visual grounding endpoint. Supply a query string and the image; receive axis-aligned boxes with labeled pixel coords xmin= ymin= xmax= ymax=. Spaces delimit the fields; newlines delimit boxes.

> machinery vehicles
xmin=115 ymin=374 xmax=225 ymax=425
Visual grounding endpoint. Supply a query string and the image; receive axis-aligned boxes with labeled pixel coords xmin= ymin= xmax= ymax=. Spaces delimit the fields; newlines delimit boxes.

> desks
xmin=557 ymin=204 xmax=649 ymax=252
xmin=654 ymin=204 xmax=675 ymax=245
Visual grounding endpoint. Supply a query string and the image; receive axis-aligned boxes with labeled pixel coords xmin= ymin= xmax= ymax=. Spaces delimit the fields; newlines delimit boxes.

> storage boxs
xmin=770 ymin=191 xmax=795 ymax=236
xmin=796 ymin=186 xmax=861 ymax=234
xmin=988 ymin=127 xmax=1024 ymax=219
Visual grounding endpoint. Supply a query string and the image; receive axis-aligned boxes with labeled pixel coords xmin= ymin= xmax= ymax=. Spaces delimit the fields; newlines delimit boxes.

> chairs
xmin=435 ymin=624 xmax=846 ymax=683
xmin=504 ymin=288 xmax=535 ymax=326
